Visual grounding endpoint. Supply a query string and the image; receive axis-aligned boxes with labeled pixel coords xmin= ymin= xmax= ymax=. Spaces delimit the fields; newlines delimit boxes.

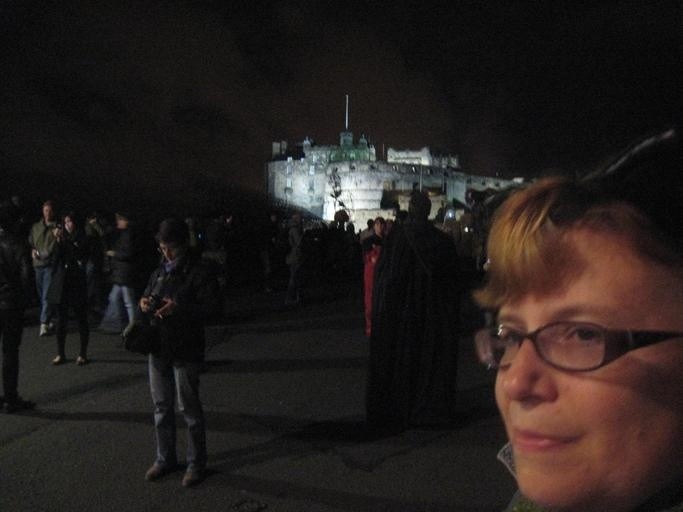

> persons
xmin=366 ymin=191 xmax=463 ymax=428
xmin=2 ymin=194 xmax=235 ymax=413
xmin=138 ymin=222 xmax=219 ymax=490
xmin=283 ymin=191 xmax=474 ymax=310
xmin=481 ymin=173 xmax=682 ymax=510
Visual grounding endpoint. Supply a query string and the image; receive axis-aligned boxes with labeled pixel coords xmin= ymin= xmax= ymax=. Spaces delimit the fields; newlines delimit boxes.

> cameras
xmin=148 ymin=295 xmax=164 ymax=312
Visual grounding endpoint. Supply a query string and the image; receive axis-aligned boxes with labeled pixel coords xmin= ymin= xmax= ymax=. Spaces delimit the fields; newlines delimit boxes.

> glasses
xmin=469 ymin=321 xmax=683 ymax=372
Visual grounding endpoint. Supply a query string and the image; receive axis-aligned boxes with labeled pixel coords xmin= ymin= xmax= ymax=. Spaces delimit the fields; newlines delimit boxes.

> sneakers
xmin=146 ymin=457 xmax=209 ymax=485
xmin=39 ymin=324 xmax=48 ymax=336
